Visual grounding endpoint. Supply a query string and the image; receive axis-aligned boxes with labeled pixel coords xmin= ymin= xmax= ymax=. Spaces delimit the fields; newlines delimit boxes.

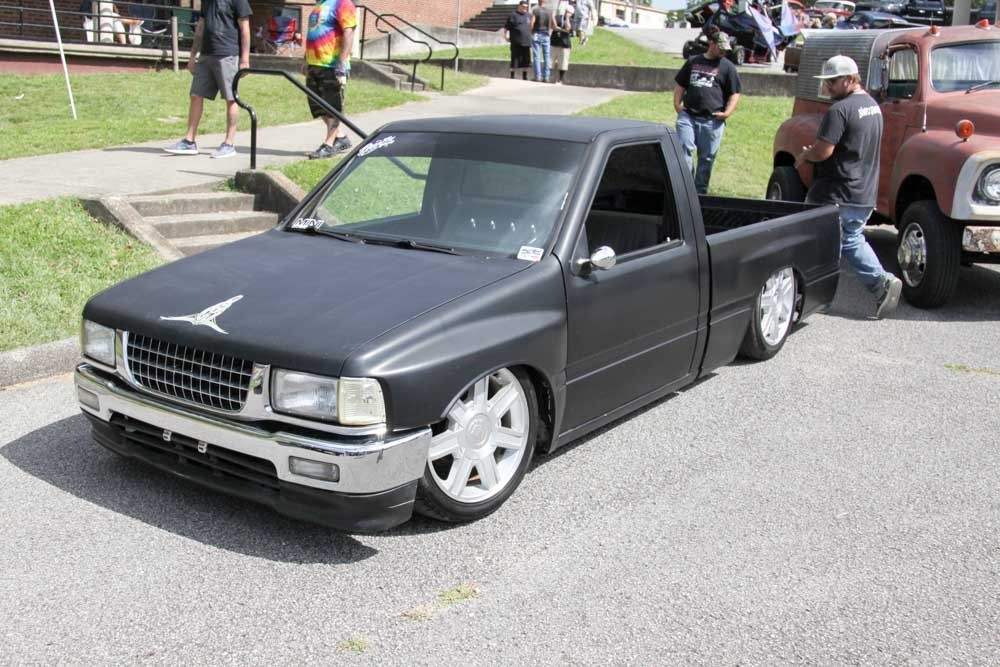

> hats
xmin=712 ymin=31 xmax=733 ymax=50
xmin=565 ymin=5 xmax=576 ymax=14
xmin=811 ymin=54 xmax=856 ymax=81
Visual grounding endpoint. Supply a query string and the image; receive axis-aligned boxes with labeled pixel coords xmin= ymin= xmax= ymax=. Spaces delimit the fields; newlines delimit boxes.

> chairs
xmin=83 ymin=3 xmax=303 ymax=56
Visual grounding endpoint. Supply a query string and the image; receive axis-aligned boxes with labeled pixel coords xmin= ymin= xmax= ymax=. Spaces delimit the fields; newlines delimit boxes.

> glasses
xmin=823 ymin=78 xmax=838 ymax=85
xmin=521 ymin=5 xmax=528 ymax=9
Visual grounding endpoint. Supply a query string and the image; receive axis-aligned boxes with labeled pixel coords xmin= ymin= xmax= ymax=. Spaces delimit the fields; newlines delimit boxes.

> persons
xmin=499 ymin=0 xmax=574 ymax=85
xmin=794 ymin=11 xmax=872 ymax=30
xmin=673 ymin=31 xmax=743 ymax=193
xmin=112 ymin=5 xmax=145 ymax=45
xmin=163 ymin=0 xmax=252 ymax=159
xmin=306 ymin=0 xmax=358 ymax=159
xmin=794 ymin=54 xmax=902 ymax=321
xmin=568 ymin=0 xmax=598 ymax=46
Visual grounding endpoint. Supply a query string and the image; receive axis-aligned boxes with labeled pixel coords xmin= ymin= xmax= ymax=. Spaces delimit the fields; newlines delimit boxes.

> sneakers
xmin=307 ymin=143 xmax=337 ymax=159
xmin=161 ymin=139 xmax=200 ymax=155
xmin=208 ymin=142 xmax=237 ymax=160
xmin=334 ymin=136 xmax=353 ymax=153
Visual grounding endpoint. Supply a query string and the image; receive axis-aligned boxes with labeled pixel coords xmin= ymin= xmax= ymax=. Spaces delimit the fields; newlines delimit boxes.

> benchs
xmin=888 ymin=79 xmax=979 ymax=97
xmin=442 ymin=197 xmax=663 ymax=253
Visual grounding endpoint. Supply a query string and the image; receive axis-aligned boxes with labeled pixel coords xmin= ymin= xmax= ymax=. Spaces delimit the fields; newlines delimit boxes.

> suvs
xmin=764 ymin=18 xmax=1000 ymax=310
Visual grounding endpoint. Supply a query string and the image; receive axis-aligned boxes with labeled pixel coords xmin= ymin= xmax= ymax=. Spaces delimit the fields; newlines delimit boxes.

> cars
xmin=763 ymin=1 xmax=1000 ymax=30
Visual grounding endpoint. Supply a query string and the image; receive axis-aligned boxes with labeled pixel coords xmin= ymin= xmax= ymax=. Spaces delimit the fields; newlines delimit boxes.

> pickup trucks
xmin=74 ymin=111 xmax=843 ymax=537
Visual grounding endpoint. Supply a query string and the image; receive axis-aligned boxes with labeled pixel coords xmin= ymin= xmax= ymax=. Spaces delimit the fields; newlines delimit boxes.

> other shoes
xmin=872 ymin=276 xmax=904 ymax=317
xmin=532 ymin=78 xmax=542 ymax=82
xmin=544 ymin=79 xmax=551 ymax=83
